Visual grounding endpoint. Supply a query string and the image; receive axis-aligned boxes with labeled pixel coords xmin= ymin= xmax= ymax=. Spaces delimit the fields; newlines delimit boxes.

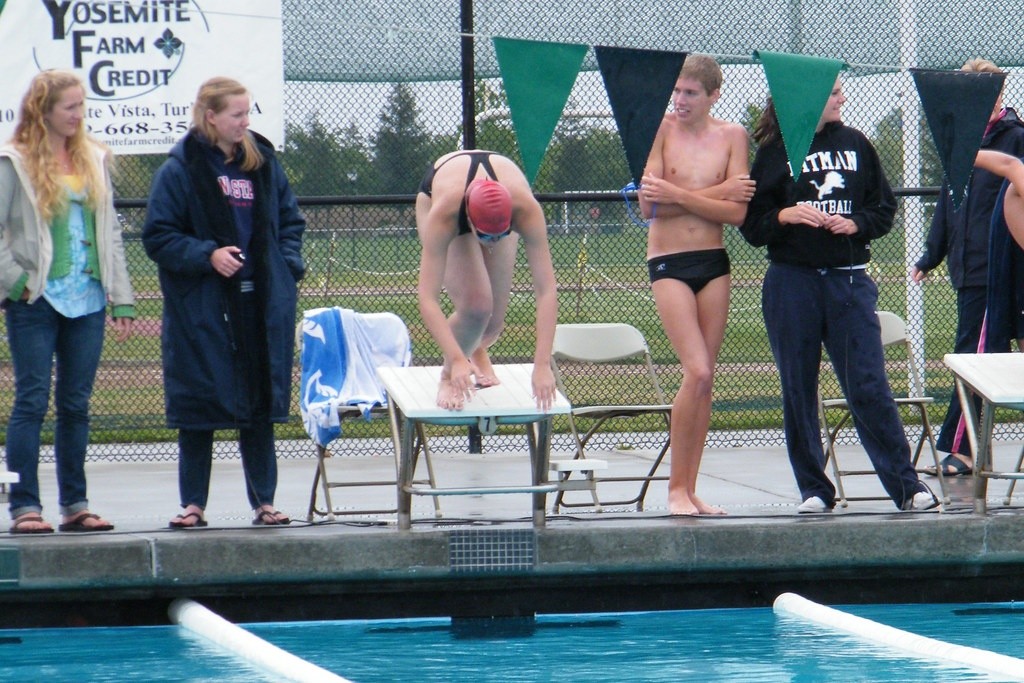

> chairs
xmin=817 ymin=310 xmax=955 ymax=507
xmin=295 ymin=307 xmax=443 ymax=523
xmin=549 ymin=322 xmax=678 ymax=515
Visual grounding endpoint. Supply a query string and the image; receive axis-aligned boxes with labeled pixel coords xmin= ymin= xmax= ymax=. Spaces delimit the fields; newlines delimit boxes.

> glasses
xmin=622 ymin=182 xmax=656 ymax=228
xmin=471 ymin=222 xmax=512 ymax=240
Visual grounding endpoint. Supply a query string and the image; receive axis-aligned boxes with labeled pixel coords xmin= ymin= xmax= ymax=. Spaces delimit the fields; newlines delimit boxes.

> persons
xmin=973 ymin=150 xmax=1023 ymax=359
xmin=0 ymin=69 xmax=138 ymax=534
xmin=907 ymin=58 xmax=1023 ymax=480
xmin=637 ymin=55 xmax=757 ymax=514
xmin=415 ymin=149 xmax=562 ymax=410
xmin=141 ymin=75 xmax=304 ymax=528
xmin=741 ymin=72 xmax=935 ymax=514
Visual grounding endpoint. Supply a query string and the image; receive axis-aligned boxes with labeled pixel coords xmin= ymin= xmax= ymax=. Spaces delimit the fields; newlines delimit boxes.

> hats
xmin=468 ymin=178 xmax=512 ymax=234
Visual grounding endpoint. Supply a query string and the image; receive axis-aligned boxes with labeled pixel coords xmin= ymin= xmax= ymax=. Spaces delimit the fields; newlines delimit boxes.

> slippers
xmin=169 ymin=513 xmax=207 ymax=527
xmin=928 ymin=454 xmax=972 ymax=475
xmin=59 ymin=513 xmax=114 ymax=531
xmin=10 ymin=517 xmax=54 ymax=533
xmin=252 ymin=511 xmax=289 ymax=525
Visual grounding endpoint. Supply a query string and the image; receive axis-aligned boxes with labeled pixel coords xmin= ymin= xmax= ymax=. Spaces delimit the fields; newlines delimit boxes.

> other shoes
xmin=904 ymin=492 xmax=942 ymax=511
xmin=797 ymin=496 xmax=834 ymax=513
